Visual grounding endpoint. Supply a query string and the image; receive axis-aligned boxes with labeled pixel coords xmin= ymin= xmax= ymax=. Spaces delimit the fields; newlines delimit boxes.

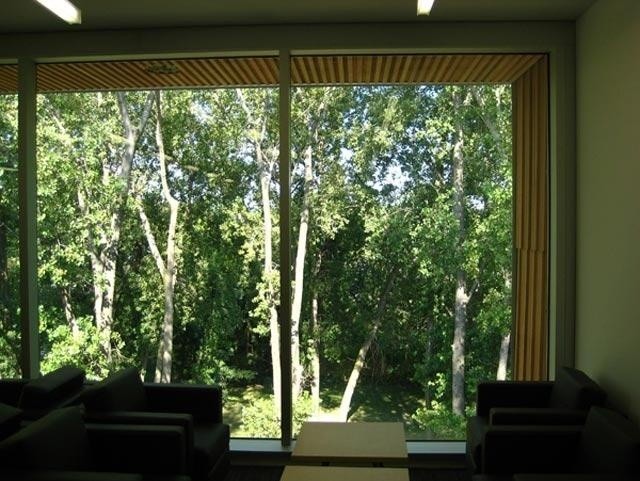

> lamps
xmin=416 ymin=0 xmax=434 ymax=16
xmin=35 ymin=0 xmax=83 ymax=25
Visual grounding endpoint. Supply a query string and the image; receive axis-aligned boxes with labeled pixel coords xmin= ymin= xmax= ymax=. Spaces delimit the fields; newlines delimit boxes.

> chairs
xmin=466 ymin=366 xmax=640 ymax=481
xmin=0 ymin=366 xmax=232 ymax=481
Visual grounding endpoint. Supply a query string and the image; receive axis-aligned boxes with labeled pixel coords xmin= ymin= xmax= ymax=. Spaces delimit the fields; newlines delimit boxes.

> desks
xmin=280 ymin=421 xmax=411 ymax=481
